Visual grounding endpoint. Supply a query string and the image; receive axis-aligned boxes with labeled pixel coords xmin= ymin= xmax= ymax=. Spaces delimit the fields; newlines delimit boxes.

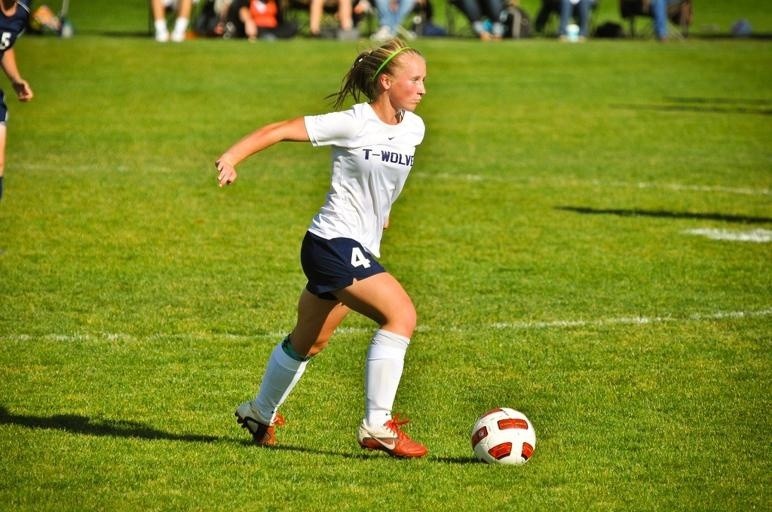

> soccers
xmin=471 ymin=407 xmax=536 ymax=465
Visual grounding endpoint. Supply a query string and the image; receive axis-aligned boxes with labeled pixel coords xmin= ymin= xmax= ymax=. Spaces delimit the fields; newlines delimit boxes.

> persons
xmin=553 ymin=0 xmax=596 ymax=43
xmin=215 ymin=38 xmax=428 ymax=458
xmin=649 ymin=0 xmax=679 ymax=43
xmin=0 ymin=0 xmax=34 ymax=199
xmin=150 ymin=0 xmax=427 ymax=42
xmin=449 ymin=0 xmax=507 ymax=40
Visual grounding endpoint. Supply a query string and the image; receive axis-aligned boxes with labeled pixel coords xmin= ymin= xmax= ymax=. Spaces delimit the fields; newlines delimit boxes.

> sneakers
xmin=357 ymin=416 xmax=428 ymax=458
xmin=235 ymin=402 xmax=285 ymax=447
xmin=154 ymin=18 xmax=188 ymax=42
